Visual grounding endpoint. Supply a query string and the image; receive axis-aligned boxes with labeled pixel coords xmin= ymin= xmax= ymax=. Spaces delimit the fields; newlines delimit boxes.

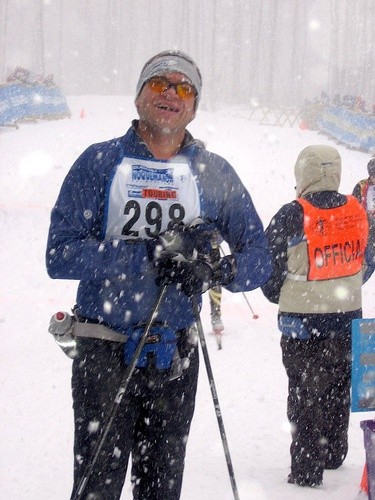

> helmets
xmin=368 ymin=159 xmax=375 ymax=184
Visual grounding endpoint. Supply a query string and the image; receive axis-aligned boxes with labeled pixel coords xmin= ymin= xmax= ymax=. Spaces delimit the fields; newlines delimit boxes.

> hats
xmin=136 ymin=51 xmax=201 ymax=104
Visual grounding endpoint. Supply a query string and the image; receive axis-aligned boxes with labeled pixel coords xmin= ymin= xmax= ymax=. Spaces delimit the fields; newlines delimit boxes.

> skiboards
xmin=215 ymin=327 xmax=223 ymax=351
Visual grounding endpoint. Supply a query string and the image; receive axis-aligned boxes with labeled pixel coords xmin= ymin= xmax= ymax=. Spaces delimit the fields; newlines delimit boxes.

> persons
xmin=351 ymin=159 xmax=375 ymax=285
xmin=260 ymin=145 xmax=375 ymax=488
xmin=209 ymin=243 xmax=224 ymax=333
xmin=45 ymin=50 xmax=275 ymax=500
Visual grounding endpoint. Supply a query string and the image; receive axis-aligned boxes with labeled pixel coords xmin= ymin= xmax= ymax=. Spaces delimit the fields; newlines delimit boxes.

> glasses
xmin=147 ymin=77 xmax=196 ymax=101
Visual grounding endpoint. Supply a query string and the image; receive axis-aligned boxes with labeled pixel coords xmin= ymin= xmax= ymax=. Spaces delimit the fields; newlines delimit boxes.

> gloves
xmin=146 ymin=219 xmax=215 ymax=264
xmin=154 ymin=251 xmax=238 ymax=297
